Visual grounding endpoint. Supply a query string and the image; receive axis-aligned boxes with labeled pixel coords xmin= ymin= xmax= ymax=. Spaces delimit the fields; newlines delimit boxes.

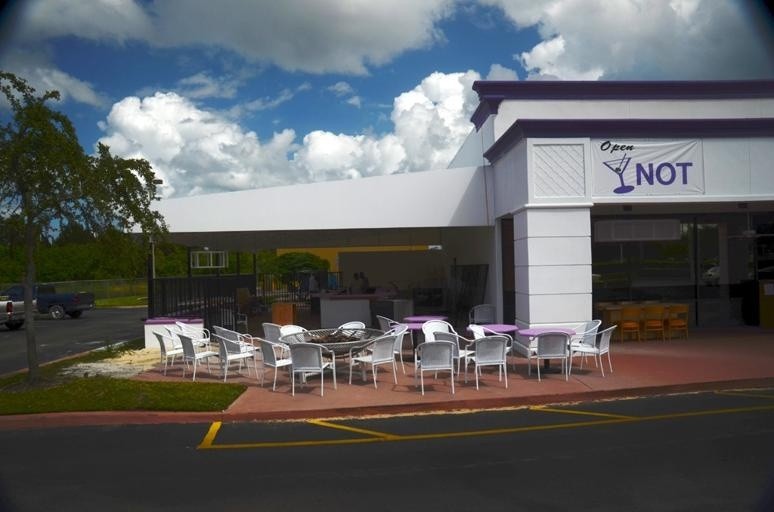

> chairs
xmin=587 ymin=300 xmax=692 ymax=346
xmin=146 ymin=279 xmax=617 ymax=397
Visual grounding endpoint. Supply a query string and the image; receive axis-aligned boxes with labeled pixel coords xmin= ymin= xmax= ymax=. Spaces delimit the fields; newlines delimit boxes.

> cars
xmin=702 ymin=266 xmax=720 ymax=286
xmin=746 ymin=266 xmax=773 ymax=281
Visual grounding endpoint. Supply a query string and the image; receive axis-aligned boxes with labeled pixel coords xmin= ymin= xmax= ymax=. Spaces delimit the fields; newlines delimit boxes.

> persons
xmin=309 ymin=268 xmax=320 ymax=291
xmin=350 ymin=270 xmax=369 ymax=294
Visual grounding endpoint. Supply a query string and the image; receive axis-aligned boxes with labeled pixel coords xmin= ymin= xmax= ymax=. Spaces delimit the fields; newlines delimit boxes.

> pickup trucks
xmin=0 ymin=296 xmax=39 ymax=330
xmin=0 ymin=282 xmax=96 ymax=322
